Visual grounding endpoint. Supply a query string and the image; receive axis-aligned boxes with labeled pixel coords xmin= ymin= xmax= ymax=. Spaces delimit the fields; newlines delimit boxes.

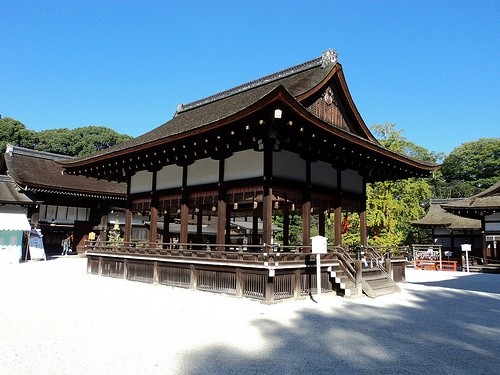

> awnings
xmin=0 ymin=204 xmax=31 ymax=232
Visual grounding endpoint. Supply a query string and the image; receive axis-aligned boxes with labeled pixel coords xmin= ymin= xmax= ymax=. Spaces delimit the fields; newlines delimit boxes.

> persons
xmin=60 ymin=231 xmax=71 ymax=256
